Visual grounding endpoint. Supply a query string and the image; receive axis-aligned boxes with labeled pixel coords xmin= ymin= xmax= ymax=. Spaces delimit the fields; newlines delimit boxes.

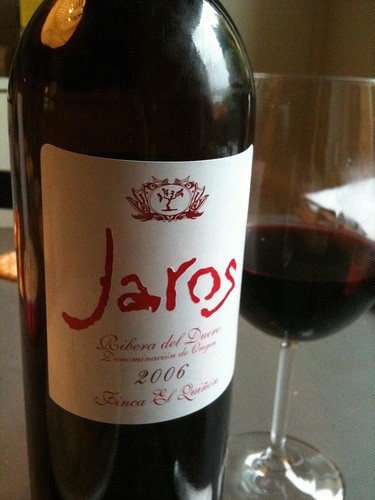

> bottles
xmin=5 ymin=0 xmax=257 ymax=500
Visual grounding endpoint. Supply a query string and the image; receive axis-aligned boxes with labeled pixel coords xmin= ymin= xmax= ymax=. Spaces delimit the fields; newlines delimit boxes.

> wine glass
xmin=215 ymin=72 xmax=374 ymax=499
